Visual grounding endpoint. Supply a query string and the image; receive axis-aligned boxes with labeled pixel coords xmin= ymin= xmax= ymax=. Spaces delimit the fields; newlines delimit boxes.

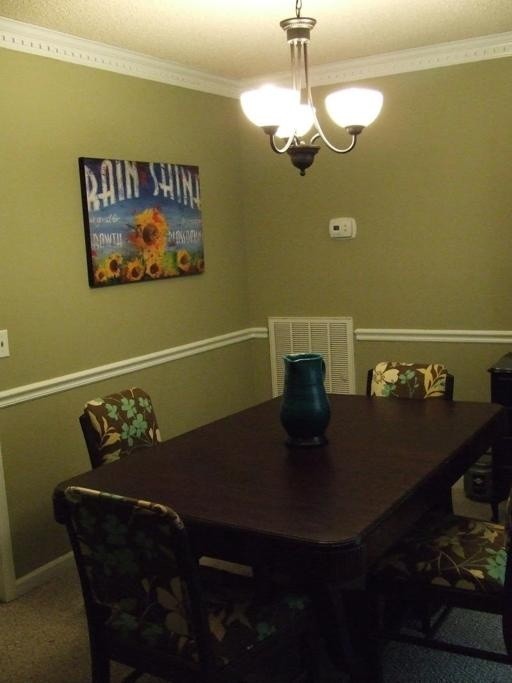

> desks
xmin=486 ymin=351 xmax=511 ymax=522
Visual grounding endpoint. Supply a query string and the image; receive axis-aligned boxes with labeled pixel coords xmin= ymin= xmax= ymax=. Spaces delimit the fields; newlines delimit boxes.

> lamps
xmin=239 ymin=0 xmax=384 ymax=176
